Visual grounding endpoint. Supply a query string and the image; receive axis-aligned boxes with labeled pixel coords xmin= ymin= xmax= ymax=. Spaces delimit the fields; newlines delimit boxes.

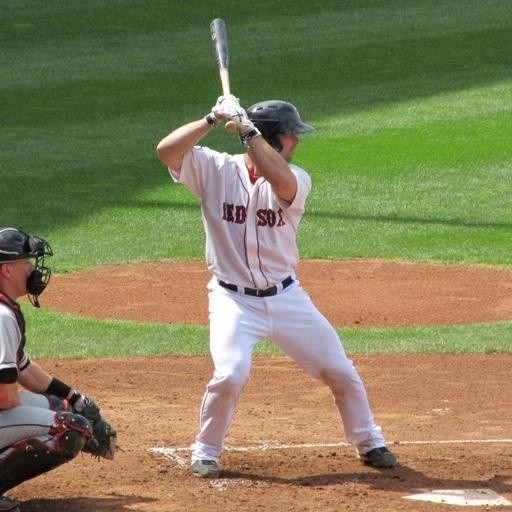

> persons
xmin=0 ymin=228 xmax=117 ymax=512
xmin=156 ymin=95 xmax=395 ymax=478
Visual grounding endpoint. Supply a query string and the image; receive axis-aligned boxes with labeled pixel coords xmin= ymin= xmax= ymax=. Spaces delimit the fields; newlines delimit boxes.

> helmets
xmin=239 ymin=100 xmax=315 ymax=147
xmin=0 ymin=228 xmax=53 ymax=263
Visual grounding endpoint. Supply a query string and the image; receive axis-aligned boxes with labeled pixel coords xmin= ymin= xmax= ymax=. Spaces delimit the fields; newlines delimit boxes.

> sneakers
xmin=191 ymin=460 xmax=219 ymax=478
xmin=0 ymin=496 xmax=17 ymax=511
xmin=358 ymin=447 xmax=396 ymax=467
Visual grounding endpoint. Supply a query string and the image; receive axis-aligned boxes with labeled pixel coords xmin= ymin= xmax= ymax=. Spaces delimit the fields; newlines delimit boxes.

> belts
xmin=219 ymin=276 xmax=295 ymax=296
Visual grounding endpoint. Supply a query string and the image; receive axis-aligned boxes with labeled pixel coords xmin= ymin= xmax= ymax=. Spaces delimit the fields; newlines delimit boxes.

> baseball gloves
xmin=69 ymin=394 xmax=119 ymax=460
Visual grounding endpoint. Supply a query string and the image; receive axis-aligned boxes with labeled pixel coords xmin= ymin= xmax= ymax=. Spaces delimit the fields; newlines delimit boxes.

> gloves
xmin=203 ymin=94 xmax=262 ymax=145
xmin=69 ymin=391 xmax=101 ymax=421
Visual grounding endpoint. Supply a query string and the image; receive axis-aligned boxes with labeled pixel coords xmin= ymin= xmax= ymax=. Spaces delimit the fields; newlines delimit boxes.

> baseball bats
xmin=209 ymin=18 xmax=238 ymax=134
